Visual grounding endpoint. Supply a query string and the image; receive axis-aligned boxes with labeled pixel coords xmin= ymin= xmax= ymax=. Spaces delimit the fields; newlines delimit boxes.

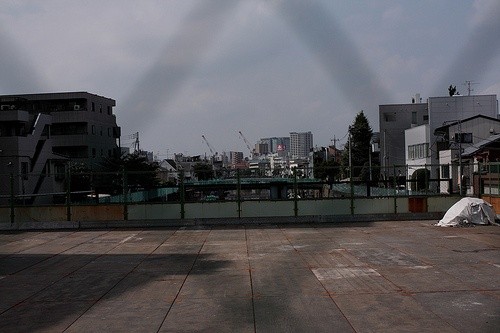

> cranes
xmin=201 ymin=134 xmax=217 ymax=163
xmin=238 ymin=131 xmax=260 ymax=161
